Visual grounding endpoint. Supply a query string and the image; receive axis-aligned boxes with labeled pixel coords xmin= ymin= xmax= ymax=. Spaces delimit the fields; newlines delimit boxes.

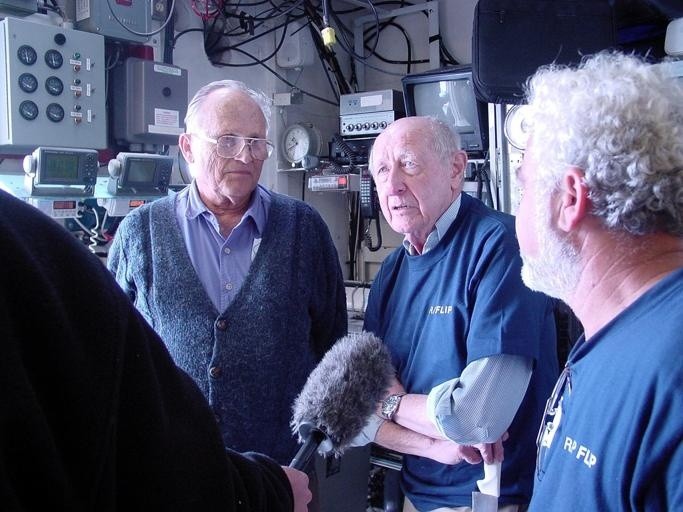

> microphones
xmin=289 ymin=330 xmax=396 ymax=471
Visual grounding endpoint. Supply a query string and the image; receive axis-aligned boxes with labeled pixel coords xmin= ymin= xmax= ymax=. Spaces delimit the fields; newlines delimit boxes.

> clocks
xmin=281 ymin=123 xmax=317 ymax=168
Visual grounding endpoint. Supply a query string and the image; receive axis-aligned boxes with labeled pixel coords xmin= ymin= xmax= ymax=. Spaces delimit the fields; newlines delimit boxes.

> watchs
xmin=381 ymin=392 xmax=406 ymax=421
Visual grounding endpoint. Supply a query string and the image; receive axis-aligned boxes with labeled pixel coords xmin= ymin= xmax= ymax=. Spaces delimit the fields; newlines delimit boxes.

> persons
xmin=0 ymin=190 xmax=314 ymax=511
xmin=359 ymin=116 xmax=558 ymax=512
xmin=515 ymin=50 xmax=683 ymax=512
xmin=106 ymin=78 xmax=347 ymax=512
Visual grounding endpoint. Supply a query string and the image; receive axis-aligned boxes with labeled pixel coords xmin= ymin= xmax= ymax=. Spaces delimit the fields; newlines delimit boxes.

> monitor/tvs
xmin=401 ymin=63 xmax=488 ymax=151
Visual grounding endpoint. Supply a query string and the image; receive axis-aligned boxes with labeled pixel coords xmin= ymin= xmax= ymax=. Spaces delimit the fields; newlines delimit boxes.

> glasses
xmin=189 ymin=135 xmax=276 ymax=162
